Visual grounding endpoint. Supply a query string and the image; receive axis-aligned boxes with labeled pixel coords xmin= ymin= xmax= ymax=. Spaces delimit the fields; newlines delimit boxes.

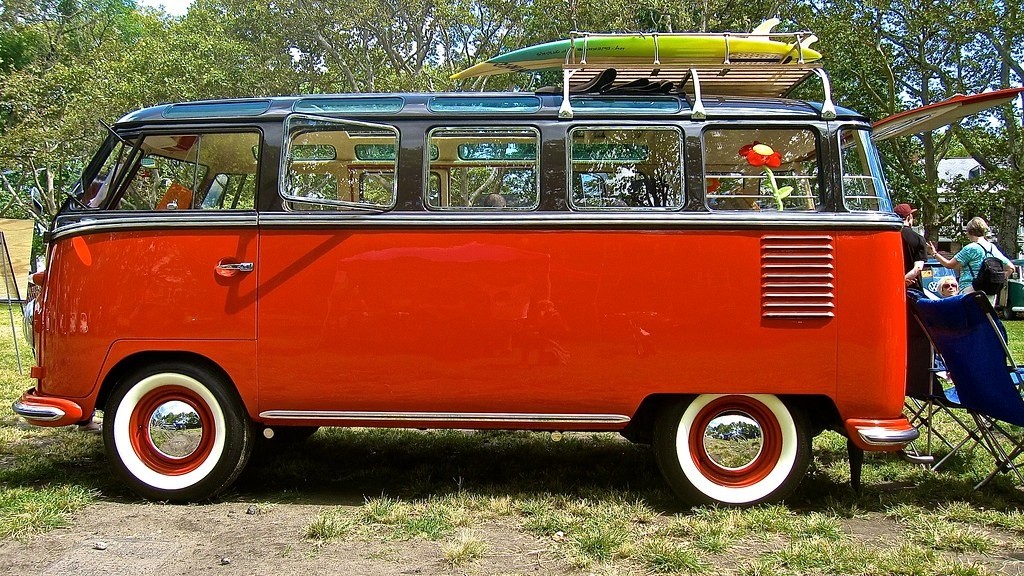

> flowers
xmin=738 ymin=141 xmax=794 ymax=212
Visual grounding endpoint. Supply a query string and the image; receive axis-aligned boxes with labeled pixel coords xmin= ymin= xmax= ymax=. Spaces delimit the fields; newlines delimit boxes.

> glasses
xmin=942 ymin=283 xmax=957 ymax=288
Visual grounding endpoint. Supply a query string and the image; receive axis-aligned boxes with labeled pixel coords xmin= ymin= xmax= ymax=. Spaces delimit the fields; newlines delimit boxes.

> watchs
xmin=932 ymin=252 xmax=938 ymax=259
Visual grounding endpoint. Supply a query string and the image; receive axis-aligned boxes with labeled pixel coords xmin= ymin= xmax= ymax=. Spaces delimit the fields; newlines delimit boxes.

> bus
xmin=10 ymin=25 xmax=1024 ymax=515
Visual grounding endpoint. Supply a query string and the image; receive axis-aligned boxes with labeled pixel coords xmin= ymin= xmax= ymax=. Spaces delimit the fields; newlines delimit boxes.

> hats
xmin=893 ymin=203 xmax=917 ymax=218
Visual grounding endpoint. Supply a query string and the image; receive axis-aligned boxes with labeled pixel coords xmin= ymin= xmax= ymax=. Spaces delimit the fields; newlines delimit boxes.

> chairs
xmin=916 ymin=292 xmax=1024 ymax=491
xmin=904 ymin=286 xmax=1023 ymax=478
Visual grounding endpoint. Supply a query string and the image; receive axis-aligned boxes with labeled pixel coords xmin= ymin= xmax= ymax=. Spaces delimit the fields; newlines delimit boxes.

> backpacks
xmin=968 ymin=242 xmax=1005 ymax=296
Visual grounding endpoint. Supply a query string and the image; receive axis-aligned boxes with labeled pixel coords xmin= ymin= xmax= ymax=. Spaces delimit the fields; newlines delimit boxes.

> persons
xmin=937 ymin=274 xmax=959 ymax=299
xmin=893 ymin=203 xmax=927 ymax=294
xmin=927 ymin=216 xmax=1016 ymax=310
